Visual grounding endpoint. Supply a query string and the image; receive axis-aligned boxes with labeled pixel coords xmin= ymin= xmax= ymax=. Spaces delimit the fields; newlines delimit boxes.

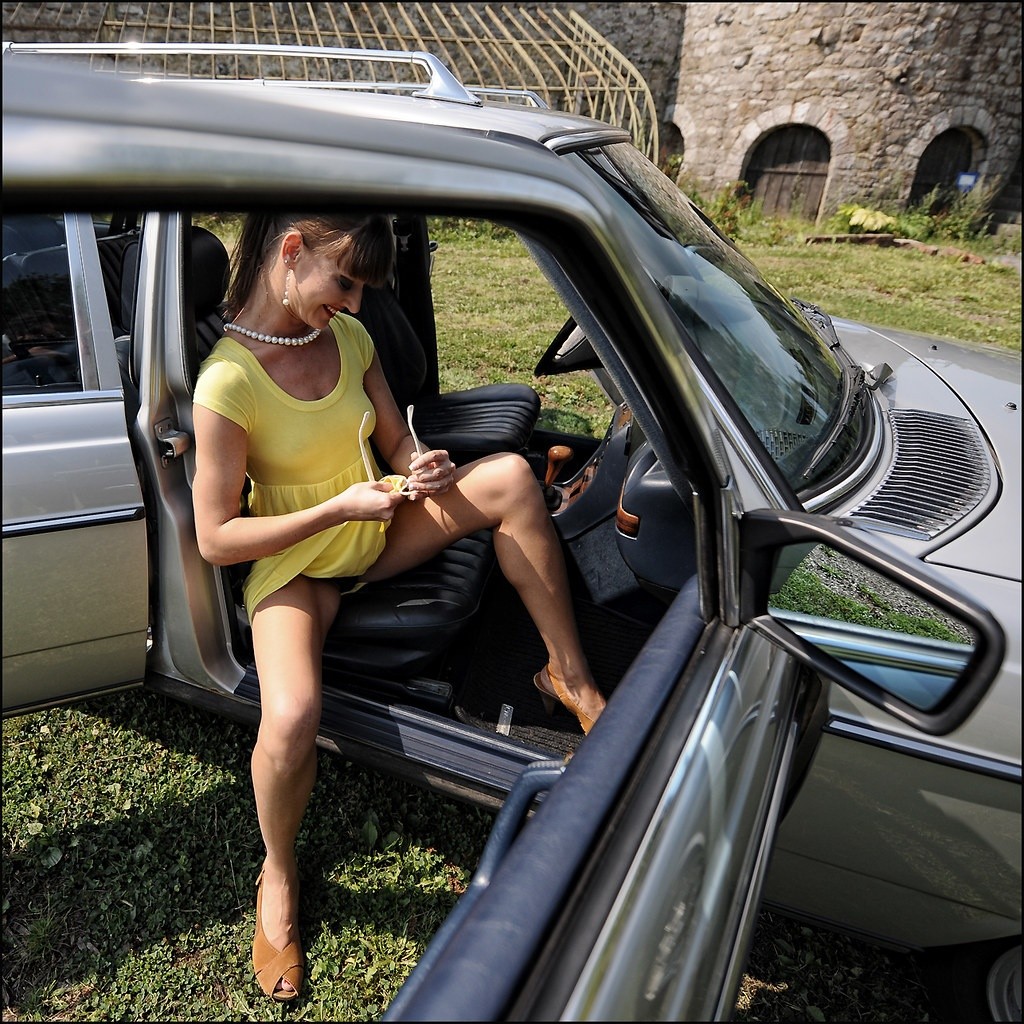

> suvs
xmin=0 ymin=44 xmax=1024 ymax=1023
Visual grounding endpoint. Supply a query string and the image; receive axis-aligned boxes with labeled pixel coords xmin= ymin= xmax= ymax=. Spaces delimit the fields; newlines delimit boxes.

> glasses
xmin=358 ymin=403 xmax=423 ymax=498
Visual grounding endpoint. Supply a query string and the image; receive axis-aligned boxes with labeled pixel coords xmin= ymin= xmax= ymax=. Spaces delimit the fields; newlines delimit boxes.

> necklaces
xmin=224 ymin=323 xmax=321 ymax=345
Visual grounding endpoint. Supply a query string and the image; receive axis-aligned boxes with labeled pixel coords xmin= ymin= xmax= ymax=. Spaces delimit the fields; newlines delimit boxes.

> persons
xmin=2 ymin=318 xmax=67 ymax=365
xmin=191 ymin=191 xmax=606 ymax=999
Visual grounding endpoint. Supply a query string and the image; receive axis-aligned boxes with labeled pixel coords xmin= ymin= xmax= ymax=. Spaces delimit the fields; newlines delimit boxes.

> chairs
xmin=116 ymin=227 xmax=543 ymax=676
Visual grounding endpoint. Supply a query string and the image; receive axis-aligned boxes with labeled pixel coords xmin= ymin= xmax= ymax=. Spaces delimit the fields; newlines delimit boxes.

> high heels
xmin=533 ymin=664 xmax=597 ymax=735
xmin=251 ymin=858 xmax=305 ymax=1000
